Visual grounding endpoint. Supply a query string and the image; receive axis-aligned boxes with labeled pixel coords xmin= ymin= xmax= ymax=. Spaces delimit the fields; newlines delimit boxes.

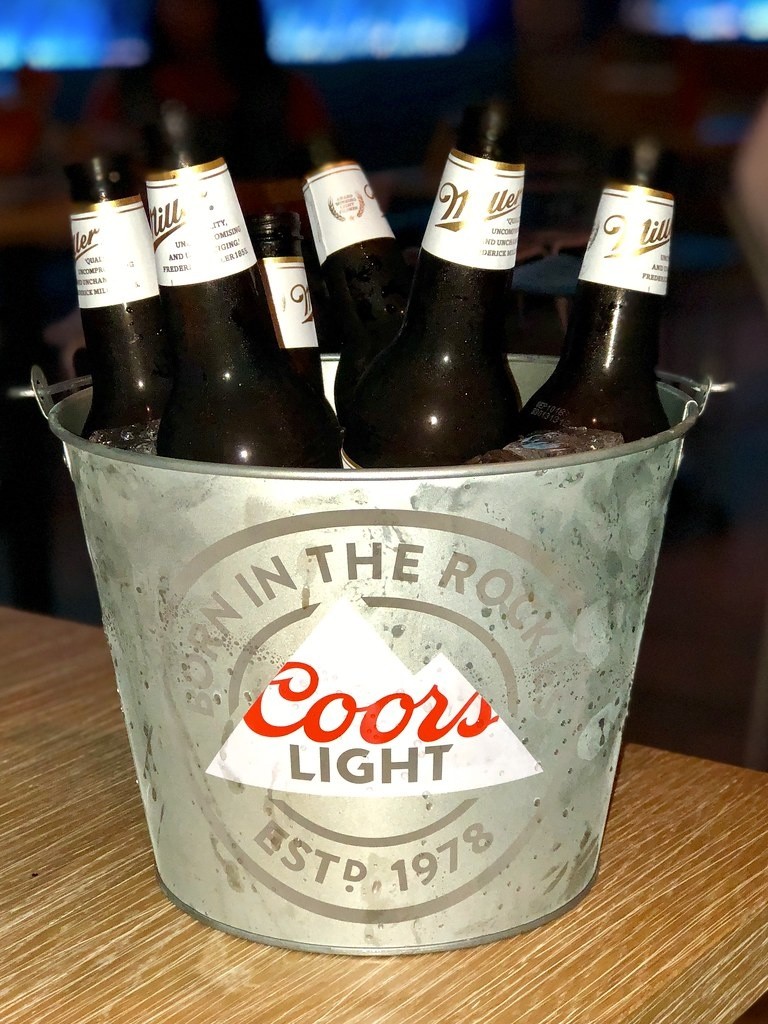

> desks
xmin=0 ymin=605 xmax=768 ymax=1024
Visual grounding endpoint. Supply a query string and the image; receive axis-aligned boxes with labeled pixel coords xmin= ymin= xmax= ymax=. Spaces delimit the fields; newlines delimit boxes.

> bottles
xmin=345 ymin=90 xmax=526 ymax=468
xmin=138 ymin=116 xmax=344 ymax=472
xmin=56 ymin=125 xmax=178 ymax=463
xmin=244 ymin=204 xmax=340 ymax=468
xmin=296 ymin=126 xmax=416 ymax=409
xmin=503 ymin=134 xmax=678 ymax=448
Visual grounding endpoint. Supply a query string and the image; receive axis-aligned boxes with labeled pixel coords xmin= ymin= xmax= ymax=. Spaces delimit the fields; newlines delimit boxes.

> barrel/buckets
xmin=25 ymin=353 xmax=742 ymax=957
